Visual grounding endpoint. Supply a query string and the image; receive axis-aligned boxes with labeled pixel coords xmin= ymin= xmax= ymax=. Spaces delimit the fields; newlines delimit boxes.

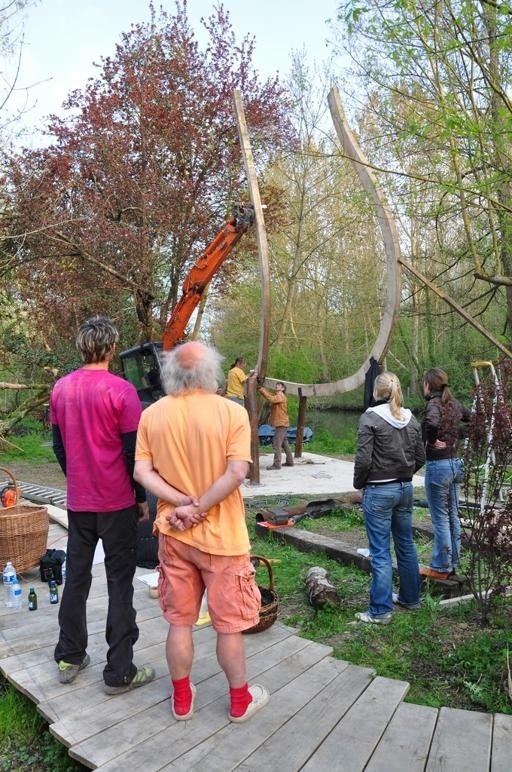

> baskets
xmin=0 ymin=467 xmax=50 ymax=579
xmin=241 ymin=554 xmax=280 ymax=635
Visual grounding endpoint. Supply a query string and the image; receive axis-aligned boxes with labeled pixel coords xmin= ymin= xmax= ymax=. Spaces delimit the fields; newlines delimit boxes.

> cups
xmin=148 ymin=584 xmax=161 ymax=599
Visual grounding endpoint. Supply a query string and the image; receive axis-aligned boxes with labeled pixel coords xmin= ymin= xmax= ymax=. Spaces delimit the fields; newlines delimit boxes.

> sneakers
xmin=355 ymin=610 xmax=394 ymax=626
xmin=57 ymin=653 xmax=91 ymax=684
xmin=105 ymin=663 xmax=155 ymax=697
xmin=390 ymin=592 xmax=422 ymax=611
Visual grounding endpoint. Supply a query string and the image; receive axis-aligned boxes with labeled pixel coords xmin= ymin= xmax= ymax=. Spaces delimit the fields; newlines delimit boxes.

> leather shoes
xmin=229 ymin=682 xmax=270 ymax=723
xmin=267 ymin=464 xmax=281 ymax=471
xmin=171 ymin=680 xmax=197 ymax=721
xmin=282 ymin=462 xmax=294 ymax=467
xmin=420 ymin=567 xmax=451 ymax=581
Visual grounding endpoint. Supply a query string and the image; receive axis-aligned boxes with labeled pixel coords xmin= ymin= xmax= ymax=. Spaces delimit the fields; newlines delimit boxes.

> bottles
xmin=2 ymin=561 xmax=18 ymax=610
xmin=47 ymin=567 xmax=57 ymax=588
xmin=50 ymin=580 xmax=59 ymax=604
xmin=12 ymin=578 xmax=23 ymax=610
xmin=27 ymin=586 xmax=38 ymax=611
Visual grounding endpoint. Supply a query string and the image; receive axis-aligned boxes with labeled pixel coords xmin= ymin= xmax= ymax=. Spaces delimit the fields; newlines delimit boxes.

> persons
xmin=49 ymin=313 xmax=157 ymax=696
xmin=223 ymin=355 xmax=258 ymax=407
xmin=132 ymin=339 xmax=272 ymax=724
xmin=256 ymin=381 xmax=294 ymax=469
xmin=351 ymin=371 xmax=427 ymax=627
xmin=140 ymin=355 xmax=164 ymax=401
xmin=420 ymin=367 xmax=474 ymax=581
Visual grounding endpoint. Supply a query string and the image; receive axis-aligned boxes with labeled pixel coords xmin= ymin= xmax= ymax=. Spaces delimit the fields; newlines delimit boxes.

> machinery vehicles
xmin=120 ymin=200 xmax=267 ymax=410
